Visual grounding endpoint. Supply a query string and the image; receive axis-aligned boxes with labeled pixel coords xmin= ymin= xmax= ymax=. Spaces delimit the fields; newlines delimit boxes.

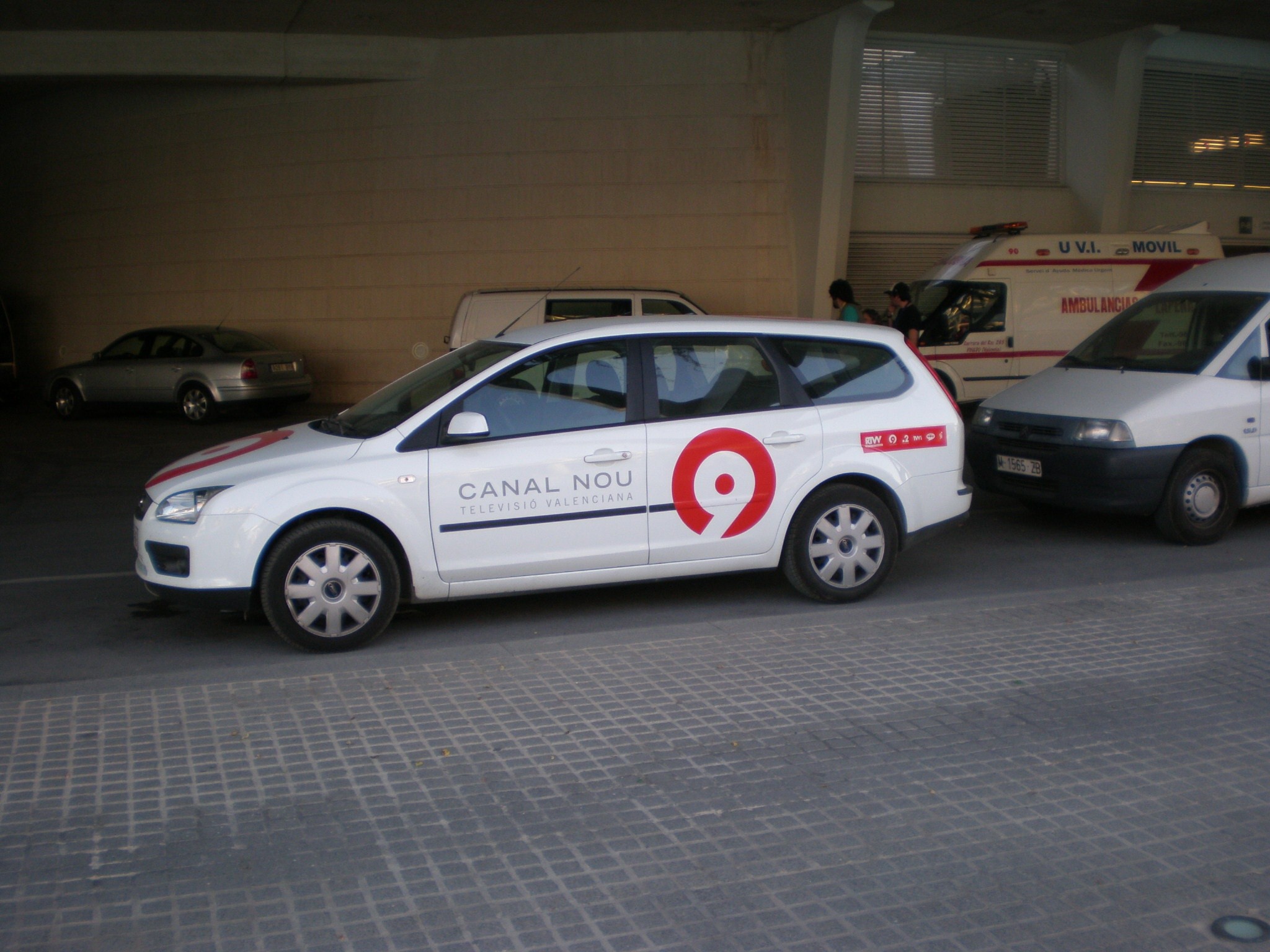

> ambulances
xmin=881 ymin=220 xmax=1234 ymax=426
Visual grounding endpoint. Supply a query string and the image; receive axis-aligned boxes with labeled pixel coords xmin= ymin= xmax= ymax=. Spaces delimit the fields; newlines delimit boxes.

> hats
xmin=884 ymin=281 xmax=908 ymax=295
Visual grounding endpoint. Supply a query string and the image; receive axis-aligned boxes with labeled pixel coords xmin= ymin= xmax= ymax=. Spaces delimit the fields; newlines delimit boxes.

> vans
xmin=443 ymin=287 xmax=774 ymax=402
xmin=965 ymin=252 xmax=1270 ymax=547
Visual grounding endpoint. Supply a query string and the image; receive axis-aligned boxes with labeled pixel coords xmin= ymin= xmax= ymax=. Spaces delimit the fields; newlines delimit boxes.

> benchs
xmin=668 ymin=368 xmax=779 ymax=417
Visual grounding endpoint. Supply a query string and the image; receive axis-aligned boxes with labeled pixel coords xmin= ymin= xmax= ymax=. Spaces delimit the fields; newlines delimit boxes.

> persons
xmin=829 ymin=278 xmax=861 ymax=322
xmin=860 ymin=308 xmax=884 ymax=326
xmin=887 ymin=282 xmax=921 ymax=349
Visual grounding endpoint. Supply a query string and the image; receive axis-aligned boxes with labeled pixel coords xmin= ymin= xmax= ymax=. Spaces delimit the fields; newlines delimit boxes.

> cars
xmin=37 ymin=324 xmax=313 ymax=428
xmin=132 ymin=308 xmax=975 ymax=655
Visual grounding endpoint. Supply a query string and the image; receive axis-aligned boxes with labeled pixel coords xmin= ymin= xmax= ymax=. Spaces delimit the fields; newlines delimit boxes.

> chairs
xmin=571 ymin=359 xmax=621 ymax=427
